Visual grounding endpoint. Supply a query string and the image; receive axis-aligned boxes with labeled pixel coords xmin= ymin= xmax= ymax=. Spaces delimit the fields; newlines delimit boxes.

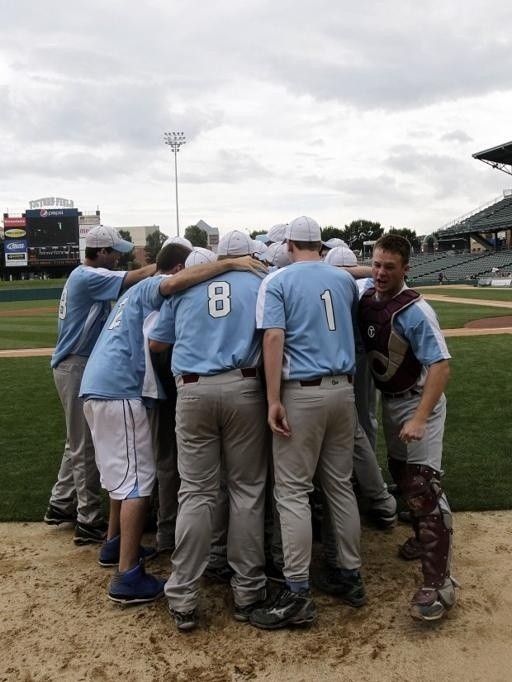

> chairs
xmin=363 ymin=197 xmax=512 ymax=281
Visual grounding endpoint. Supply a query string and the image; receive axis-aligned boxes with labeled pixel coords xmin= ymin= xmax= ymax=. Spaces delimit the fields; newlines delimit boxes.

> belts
xmin=298 ymin=373 xmax=353 ymax=388
xmin=181 ymin=364 xmax=257 ymax=385
xmin=382 ymin=385 xmax=420 ymax=400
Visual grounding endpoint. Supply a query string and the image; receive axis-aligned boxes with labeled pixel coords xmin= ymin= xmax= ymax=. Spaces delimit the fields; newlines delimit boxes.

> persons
xmin=43 ymin=218 xmax=454 ymax=631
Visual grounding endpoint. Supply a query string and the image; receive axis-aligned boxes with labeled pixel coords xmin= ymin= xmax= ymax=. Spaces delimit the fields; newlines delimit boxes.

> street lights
xmin=162 ymin=130 xmax=188 ymax=236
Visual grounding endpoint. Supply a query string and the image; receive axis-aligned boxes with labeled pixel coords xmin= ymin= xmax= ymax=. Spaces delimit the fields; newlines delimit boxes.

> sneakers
xmin=107 ymin=563 xmax=167 ymax=605
xmin=408 ymin=581 xmax=456 ymax=622
xmin=377 ymin=480 xmax=422 ymax=559
xmin=42 ymin=500 xmax=79 ymax=524
xmin=313 ymin=565 xmax=368 ymax=607
xmin=201 ymin=550 xmax=285 ymax=581
xmin=74 ymin=522 xmax=108 ymax=545
xmin=248 ymin=588 xmax=317 ymax=630
xmin=97 ymin=534 xmax=158 ymax=566
xmin=166 ymin=599 xmax=199 ymax=632
xmin=230 ymin=582 xmax=286 ymax=622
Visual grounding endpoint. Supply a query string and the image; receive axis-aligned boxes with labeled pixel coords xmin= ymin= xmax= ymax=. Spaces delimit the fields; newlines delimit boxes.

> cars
xmin=28 ymin=271 xmax=50 ymax=280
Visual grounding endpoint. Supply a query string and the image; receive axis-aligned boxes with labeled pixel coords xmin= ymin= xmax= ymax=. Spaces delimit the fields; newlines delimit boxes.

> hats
xmin=280 ymin=216 xmax=321 ymax=242
xmin=321 ymin=238 xmax=349 ymax=249
xmin=84 ymin=224 xmax=136 ymax=254
xmin=161 ymin=224 xmax=291 ymax=268
xmin=324 ymin=246 xmax=360 ymax=267
